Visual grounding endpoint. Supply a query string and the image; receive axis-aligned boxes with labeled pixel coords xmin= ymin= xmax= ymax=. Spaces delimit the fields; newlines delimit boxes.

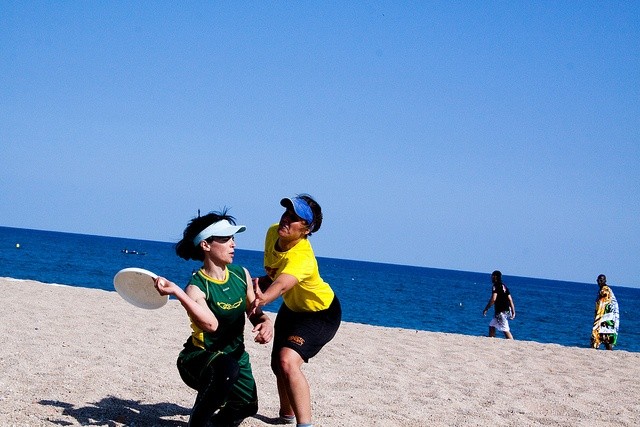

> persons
xmin=151 ymin=203 xmax=272 ymax=427
xmin=590 ymin=274 xmax=620 ymax=350
xmin=483 ymin=270 xmax=515 ymax=340
xmin=247 ymin=192 xmax=342 ymax=427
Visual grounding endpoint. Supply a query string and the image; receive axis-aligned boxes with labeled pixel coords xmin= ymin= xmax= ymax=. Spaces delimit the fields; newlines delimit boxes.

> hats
xmin=192 ymin=218 xmax=247 ymax=247
xmin=279 ymin=196 xmax=314 ymax=225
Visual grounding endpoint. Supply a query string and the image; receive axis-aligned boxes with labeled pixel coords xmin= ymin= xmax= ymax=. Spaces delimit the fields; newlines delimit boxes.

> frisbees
xmin=112 ymin=266 xmax=170 ymax=311
